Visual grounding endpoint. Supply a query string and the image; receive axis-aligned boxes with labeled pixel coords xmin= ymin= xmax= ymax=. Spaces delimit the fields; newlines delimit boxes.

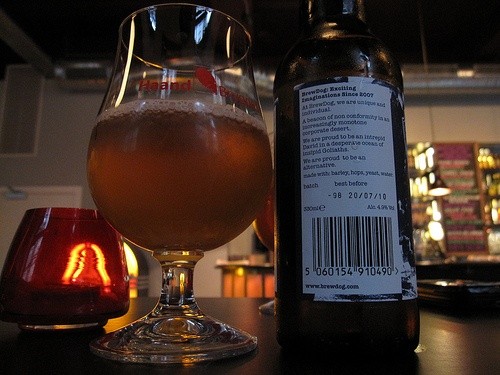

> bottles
xmin=267 ymin=0 xmax=419 ymax=359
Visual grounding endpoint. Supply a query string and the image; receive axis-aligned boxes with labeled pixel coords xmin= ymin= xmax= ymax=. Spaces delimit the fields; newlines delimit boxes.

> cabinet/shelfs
xmin=407 ymin=143 xmax=500 ymax=260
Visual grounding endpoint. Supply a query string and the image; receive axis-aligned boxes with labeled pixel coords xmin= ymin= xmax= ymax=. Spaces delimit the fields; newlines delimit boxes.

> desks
xmin=0 ymin=297 xmax=500 ymax=375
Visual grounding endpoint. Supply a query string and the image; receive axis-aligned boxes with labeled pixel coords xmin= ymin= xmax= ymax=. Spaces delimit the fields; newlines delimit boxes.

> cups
xmin=0 ymin=206 xmax=130 ymax=331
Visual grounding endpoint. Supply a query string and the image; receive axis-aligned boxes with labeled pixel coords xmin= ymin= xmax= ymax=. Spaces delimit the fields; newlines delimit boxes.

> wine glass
xmin=252 ymin=131 xmax=275 ymax=316
xmin=85 ymin=2 xmax=274 ymax=365
